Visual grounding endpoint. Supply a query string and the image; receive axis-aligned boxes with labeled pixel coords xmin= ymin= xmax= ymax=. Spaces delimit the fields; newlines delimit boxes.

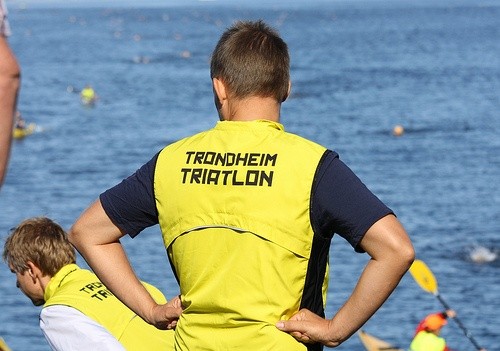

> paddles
xmin=408 ymin=259 xmax=483 ymax=351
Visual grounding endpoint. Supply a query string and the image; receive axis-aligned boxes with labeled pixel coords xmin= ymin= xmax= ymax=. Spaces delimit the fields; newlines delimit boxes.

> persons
xmin=69 ymin=19 xmax=416 ymax=351
xmin=408 ymin=310 xmax=486 ymax=351
xmin=0 ymin=0 xmax=100 ymax=351
xmin=3 ymin=217 xmax=176 ymax=351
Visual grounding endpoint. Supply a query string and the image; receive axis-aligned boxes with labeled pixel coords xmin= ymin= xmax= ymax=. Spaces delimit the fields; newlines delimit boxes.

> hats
xmin=423 ymin=313 xmax=448 ymax=332
